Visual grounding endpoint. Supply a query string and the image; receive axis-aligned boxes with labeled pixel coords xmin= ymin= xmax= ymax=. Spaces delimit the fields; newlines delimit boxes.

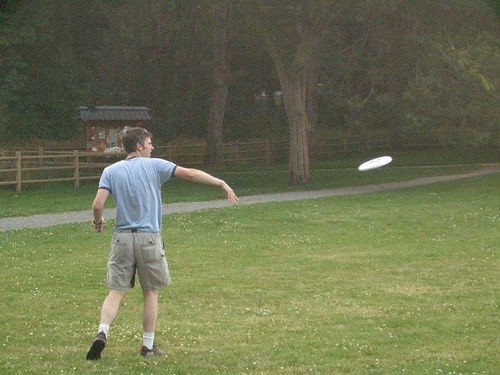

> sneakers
xmin=141 ymin=346 xmax=166 ymax=356
xmin=87 ymin=330 xmax=107 ymax=361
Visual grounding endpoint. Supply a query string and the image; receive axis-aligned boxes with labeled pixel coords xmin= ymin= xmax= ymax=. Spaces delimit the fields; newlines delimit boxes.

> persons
xmin=87 ymin=126 xmax=239 ymax=363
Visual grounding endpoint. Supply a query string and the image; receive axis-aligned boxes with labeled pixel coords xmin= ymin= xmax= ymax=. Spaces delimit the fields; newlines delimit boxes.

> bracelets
xmin=92 ymin=217 xmax=105 ymax=227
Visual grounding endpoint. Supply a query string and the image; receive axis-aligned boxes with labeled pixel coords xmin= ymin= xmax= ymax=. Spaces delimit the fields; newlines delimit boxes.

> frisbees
xmin=358 ymin=156 xmax=392 ymax=171
